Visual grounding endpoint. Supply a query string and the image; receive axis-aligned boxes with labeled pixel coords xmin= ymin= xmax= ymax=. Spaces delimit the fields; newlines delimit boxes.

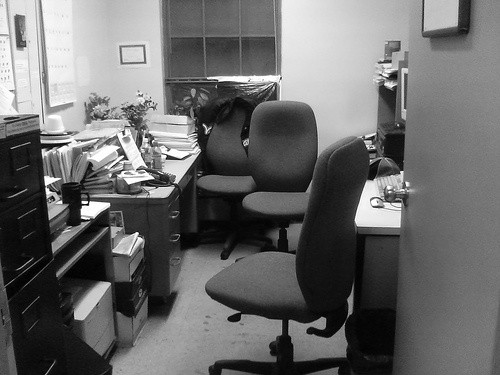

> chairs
xmin=235 ymin=100 xmax=318 ymax=264
xmin=205 ymin=137 xmax=369 ymax=375
xmin=178 ymin=101 xmax=274 ymax=261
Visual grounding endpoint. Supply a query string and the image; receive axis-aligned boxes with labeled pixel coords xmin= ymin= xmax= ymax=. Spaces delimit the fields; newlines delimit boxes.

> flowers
xmin=122 ymin=90 xmax=159 ymax=133
xmin=84 ymin=92 xmax=121 ymax=123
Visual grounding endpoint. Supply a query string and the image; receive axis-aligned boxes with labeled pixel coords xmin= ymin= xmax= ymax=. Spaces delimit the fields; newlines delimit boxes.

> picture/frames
xmin=422 ymin=1 xmax=470 ymax=37
xmin=116 ymin=40 xmax=150 ymax=69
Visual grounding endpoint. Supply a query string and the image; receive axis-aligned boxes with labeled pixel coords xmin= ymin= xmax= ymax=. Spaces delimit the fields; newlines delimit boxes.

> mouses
xmin=369 ymin=197 xmax=384 ymax=209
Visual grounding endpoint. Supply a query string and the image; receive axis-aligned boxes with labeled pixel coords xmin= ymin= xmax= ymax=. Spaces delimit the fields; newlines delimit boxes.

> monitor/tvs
xmin=399 ymin=68 xmax=408 ymax=121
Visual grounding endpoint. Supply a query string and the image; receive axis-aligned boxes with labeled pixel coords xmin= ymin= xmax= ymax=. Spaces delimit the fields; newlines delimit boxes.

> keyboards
xmin=375 ymin=174 xmax=404 ymax=202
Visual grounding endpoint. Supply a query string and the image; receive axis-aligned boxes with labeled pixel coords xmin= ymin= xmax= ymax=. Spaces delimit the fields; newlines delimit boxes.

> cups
xmin=61 ymin=181 xmax=90 ymax=226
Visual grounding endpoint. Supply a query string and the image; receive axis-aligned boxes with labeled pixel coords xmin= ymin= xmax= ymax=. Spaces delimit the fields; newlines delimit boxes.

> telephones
xmin=136 ymin=166 xmax=184 ymax=196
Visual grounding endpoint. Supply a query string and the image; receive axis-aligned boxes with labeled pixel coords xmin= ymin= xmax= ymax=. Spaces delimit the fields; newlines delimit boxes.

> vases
xmin=136 ymin=131 xmax=155 ymax=149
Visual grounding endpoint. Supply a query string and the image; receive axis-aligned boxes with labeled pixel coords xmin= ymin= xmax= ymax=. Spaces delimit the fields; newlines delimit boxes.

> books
xmin=148 ymin=115 xmax=202 ymax=155
xmin=39 ymin=134 xmax=126 ymax=194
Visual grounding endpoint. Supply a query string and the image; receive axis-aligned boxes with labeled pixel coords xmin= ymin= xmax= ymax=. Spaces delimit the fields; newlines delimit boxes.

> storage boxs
xmin=112 ymin=234 xmax=145 ymax=300
xmin=114 ymin=287 xmax=148 ymax=346
xmin=70 ymin=282 xmax=114 ymax=356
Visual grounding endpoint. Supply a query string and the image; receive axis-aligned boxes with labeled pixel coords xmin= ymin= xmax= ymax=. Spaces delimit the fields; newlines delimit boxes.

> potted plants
xmin=176 ymin=88 xmax=209 ymax=135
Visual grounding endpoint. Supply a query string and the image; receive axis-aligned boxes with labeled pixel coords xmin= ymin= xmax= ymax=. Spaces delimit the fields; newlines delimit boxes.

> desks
xmin=353 ymin=169 xmax=402 ymax=312
xmin=48 ymin=196 xmax=117 ymax=361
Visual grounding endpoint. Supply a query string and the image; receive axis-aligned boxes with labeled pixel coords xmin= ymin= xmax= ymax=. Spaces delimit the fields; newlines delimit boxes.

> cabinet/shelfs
xmin=0 ymin=129 xmax=66 ymax=374
xmin=79 ymin=196 xmax=181 ymax=303
xmin=377 ymin=40 xmax=404 ymax=157
xmin=181 ymin=150 xmax=205 ymax=232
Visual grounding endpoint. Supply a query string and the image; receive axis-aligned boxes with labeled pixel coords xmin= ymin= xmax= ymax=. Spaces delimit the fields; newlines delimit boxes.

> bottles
xmin=139 ymin=137 xmax=152 ymax=168
xmin=151 ymin=140 xmax=163 ymax=173
